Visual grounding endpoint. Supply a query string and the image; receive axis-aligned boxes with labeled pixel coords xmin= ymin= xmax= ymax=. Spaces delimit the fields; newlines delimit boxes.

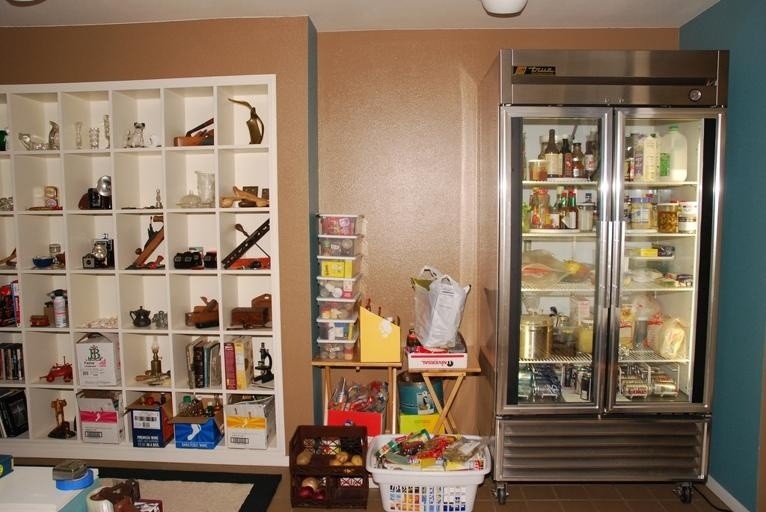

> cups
xmin=195 ymin=172 xmax=213 ymax=204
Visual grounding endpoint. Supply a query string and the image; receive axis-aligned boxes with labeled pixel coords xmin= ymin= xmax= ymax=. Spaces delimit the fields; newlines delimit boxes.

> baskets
xmin=365 ymin=433 xmax=492 ymax=512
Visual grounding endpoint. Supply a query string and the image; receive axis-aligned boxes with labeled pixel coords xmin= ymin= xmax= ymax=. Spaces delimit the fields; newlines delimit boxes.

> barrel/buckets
xmin=658 ymin=124 xmax=688 ymax=182
xmin=396 ymin=370 xmax=445 ymax=416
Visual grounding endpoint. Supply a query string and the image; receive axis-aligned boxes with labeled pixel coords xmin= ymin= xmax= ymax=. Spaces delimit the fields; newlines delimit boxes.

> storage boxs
xmin=403 ymin=342 xmax=469 ymax=374
xmin=315 ymin=211 xmax=364 ymax=361
xmin=398 ymin=414 xmax=447 ymax=436
xmin=76 ymin=390 xmax=276 ymax=450
xmin=73 ymin=331 xmax=120 ymax=386
xmin=328 ymin=404 xmax=386 ymax=434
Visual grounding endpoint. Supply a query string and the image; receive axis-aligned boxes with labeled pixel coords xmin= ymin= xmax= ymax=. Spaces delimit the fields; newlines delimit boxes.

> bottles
xmin=54 ymin=290 xmax=66 ymax=327
xmin=180 ymin=394 xmax=222 ymax=417
xmin=540 ymin=128 xmax=598 ymax=178
xmin=404 ymin=327 xmax=420 ymax=354
xmin=529 ymin=187 xmax=597 ymax=228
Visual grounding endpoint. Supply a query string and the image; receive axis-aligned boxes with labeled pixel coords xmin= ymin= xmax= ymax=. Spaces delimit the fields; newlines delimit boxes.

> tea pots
xmin=129 ymin=305 xmax=152 ymax=328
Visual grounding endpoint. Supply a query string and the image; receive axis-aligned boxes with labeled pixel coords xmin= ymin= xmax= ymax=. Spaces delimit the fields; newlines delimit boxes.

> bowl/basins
xmin=32 ymin=258 xmax=51 ymax=267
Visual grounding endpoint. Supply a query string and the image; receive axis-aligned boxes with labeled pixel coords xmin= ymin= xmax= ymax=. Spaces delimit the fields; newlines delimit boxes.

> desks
xmin=417 ymin=344 xmax=483 ymax=434
xmin=314 ymin=359 xmax=402 ymax=437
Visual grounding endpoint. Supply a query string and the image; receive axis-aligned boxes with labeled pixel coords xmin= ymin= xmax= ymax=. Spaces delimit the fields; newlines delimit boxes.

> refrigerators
xmin=480 ymin=46 xmax=731 ymax=503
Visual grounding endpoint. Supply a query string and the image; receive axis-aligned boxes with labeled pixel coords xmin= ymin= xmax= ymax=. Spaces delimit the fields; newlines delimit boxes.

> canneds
xmin=618 ymin=373 xmax=677 ymax=396
xmin=527 ymin=160 xmax=546 ymax=182
xmin=565 ymin=364 xmax=592 ymax=400
xmin=336 ymin=392 xmax=347 ymax=402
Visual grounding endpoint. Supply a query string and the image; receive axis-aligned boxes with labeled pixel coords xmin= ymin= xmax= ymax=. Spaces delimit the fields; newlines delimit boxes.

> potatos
xmin=301 ymin=477 xmax=318 ymax=490
xmin=336 ymin=452 xmax=348 ymax=463
xmin=344 ymin=462 xmax=352 ymax=473
xmin=295 ymin=450 xmax=313 ymax=466
xmin=329 ymin=460 xmax=342 ymax=467
xmin=351 ymin=455 xmax=361 ymax=464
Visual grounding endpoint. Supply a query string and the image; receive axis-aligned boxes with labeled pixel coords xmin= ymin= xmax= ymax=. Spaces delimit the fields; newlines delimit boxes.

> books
xmin=186 ymin=336 xmax=255 ymax=390
xmin=0 ymin=280 xmax=29 ymax=439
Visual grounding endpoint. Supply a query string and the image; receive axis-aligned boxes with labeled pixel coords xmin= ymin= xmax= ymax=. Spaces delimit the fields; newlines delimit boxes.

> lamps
xmin=480 ymin=1 xmax=528 ymax=16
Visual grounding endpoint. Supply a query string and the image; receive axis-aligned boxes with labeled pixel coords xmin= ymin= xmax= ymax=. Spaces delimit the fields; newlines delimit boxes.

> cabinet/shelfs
xmin=0 ymin=71 xmax=289 ymax=469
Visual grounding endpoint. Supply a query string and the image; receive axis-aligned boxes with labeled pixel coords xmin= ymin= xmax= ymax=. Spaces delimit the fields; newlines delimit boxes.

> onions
xmin=313 ymin=490 xmax=326 ymax=503
xmin=297 ymin=486 xmax=314 ymax=500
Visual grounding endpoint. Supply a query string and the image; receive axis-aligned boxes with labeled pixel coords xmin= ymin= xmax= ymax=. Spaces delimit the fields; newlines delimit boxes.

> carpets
xmin=0 ymin=462 xmax=282 ymax=511
